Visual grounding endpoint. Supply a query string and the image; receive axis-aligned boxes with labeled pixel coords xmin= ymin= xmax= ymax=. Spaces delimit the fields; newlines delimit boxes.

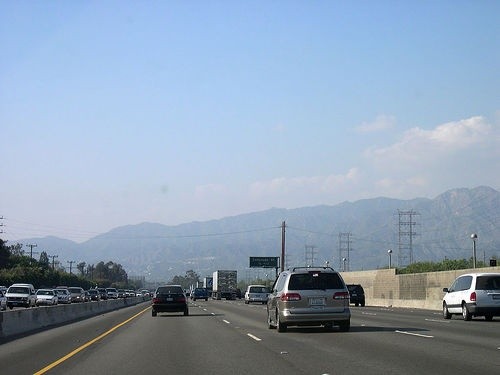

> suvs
xmin=190 ymin=288 xmax=208 ymax=302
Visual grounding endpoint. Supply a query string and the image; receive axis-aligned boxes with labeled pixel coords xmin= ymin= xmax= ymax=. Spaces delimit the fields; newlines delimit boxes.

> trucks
xmin=190 ymin=269 xmax=238 ymax=301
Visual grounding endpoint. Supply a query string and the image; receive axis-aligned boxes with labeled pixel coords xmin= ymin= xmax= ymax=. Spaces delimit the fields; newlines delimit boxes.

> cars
xmin=90 ymin=289 xmax=100 ymax=301
xmin=442 ymin=272 xmax=500 ymax=321
xmin=35 ymin=289 xmax=58 ymax=307
xmin=117 ymin=289 xmax=126 ymax=298
xmin=244 ymin=285 xmax=269 ymax=304
xmin=53 ymin=289 xmax=72 ymax=304
xmin=266 ymin=266 xmax=351 ymax=333
xmin=0 ymin=290 xmax=7 ymax=311
xmin=345 ymin=283 xmax=366 ymax=307
xmin=5 ymin=284 xmax=38 ymax=309
xmin=68 ymin=287 xmax=86 ymax=303
xmin=96 ymin=288 xmax=108 ymax=300
xmin=0 ymin=286 xmax=8 ymax=295
xmin=125 ymin=289 xmax=154 ymax=297
xmin=151 ymin=285 xmax=190 ymax=317
xmin=106 ymin=288 xmax=118 ymax=299
xmin=84 ymin=290 xmax=91 ymax=302
